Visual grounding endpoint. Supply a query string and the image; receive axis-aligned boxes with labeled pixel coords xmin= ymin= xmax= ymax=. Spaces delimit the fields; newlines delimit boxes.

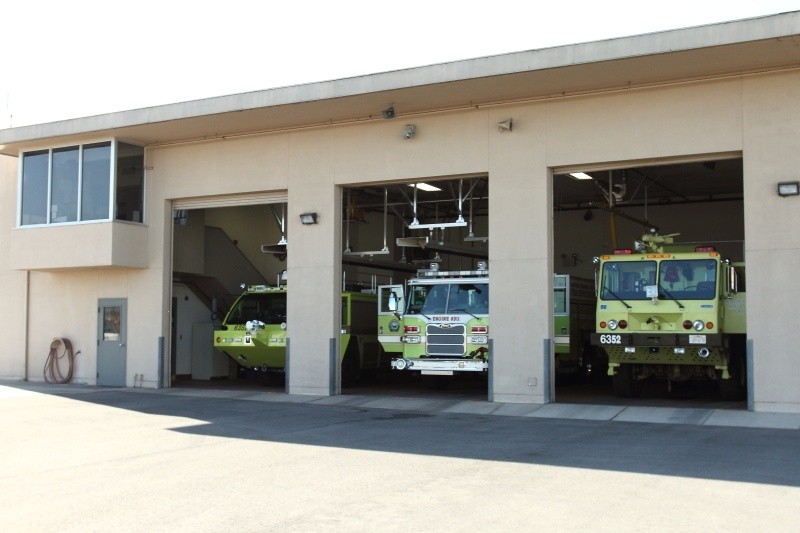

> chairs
xmin=696 ymin=281 xmax=716 ymax=292
xmin=621 ymin=272 xmax=640 ymax=292
xmin=474 ymin=289 xmax=488 ymax=308
xmin=426 ymin=298 xmax=453 ymax=311
xmin=258 ymin=296 xmax=283 ymax=316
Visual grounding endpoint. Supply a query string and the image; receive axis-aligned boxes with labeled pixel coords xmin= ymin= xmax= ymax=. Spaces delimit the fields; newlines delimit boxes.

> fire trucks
xmin=212 ymin=269 xmax=378 ymax=387
xmin=377 ymin=259 xmax=596 ymax=390
xmin=593 ymin=229 xmax=747 ymax=402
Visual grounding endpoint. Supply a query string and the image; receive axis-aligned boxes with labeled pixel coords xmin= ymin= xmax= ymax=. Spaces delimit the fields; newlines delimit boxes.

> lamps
xmin=777 ymin=181 xmax=800 ymax=197
xmin=497 ymin=117 xmax=512 ymax=133
xmin=300 ymin=213 xmax=317 ymax=225
